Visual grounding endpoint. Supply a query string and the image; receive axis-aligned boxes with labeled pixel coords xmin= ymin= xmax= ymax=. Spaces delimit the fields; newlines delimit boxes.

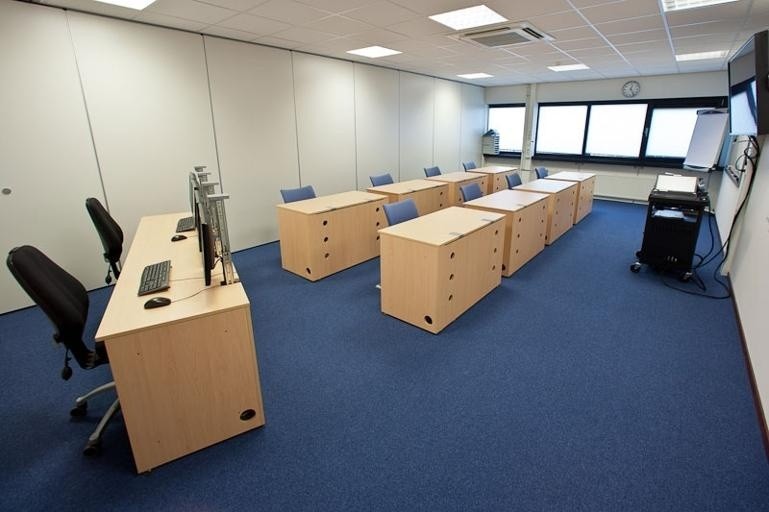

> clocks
xmin=621 ymin=80 xmax=641 ymax=98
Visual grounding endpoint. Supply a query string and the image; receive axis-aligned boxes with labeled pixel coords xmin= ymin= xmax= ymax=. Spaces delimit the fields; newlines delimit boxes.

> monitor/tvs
xmin=188 ymin=166 xmax=241 ymax=287
xmin=725 ymin=28 xmax=769 ymax=139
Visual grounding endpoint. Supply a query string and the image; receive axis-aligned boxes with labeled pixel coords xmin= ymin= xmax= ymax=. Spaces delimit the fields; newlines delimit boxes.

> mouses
xmin=700 ymin=196 xmax=707 ymax=200
xmin=144 ymin=297 xmax=171 ymax=309
xmin=171 ymin=234 xmax=187 ymax=242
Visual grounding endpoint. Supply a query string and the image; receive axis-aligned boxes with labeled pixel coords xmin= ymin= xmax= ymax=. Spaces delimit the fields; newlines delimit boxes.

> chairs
xmin=535 ymin=167 xmax=549 ymax=179
xmin=369 ymin=172 xmax=394 ymax=187
xmin=4 ymin=243 xmax=130 ymax=458
xmin=505 ymin=172 xmax=522 ymax=191
xmin=383 ymin=199 xmax=420 ymax=226
xmin=423 ymin=166 xmax=441 ymax=178
xmin=463 ymin=161 xmax=476 ymax=172
xmin=280 ymin=185 xmax=316 ymax=203
xmin=84 ymin=195 xmax=126 ymax=285
xmin=459 ymin=182 xmax=483 ymax=202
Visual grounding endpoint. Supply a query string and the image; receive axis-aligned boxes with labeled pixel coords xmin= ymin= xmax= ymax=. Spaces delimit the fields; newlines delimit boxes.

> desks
xmin=511 ymin=179 xmax=579 ymax=245
xmin=367 ymin=179 xmax=449 ymax=226
xmin=544 ymin=171 xmax=596 ymax=225
xmin=464 ymin=165 xmax=518 ymax=196
xmin=425 ymin=171 xmax=488 ymax=207
xmin=94 ymin=207 xmax=268 ymax=480
xmin=377 ymin=206 xmax=507 ymax=334
xmin=461 ymin=188 xmax=551 ymax=277
xmin=276 ymin=190 xmax=389 ymax=282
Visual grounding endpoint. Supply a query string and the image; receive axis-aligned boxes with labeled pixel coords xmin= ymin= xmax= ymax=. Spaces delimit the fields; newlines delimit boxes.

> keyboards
xmin=176 ymin=216 xmax=196 ymax=233
xmin=138 ymin=259 xmax=171 ymax=296
